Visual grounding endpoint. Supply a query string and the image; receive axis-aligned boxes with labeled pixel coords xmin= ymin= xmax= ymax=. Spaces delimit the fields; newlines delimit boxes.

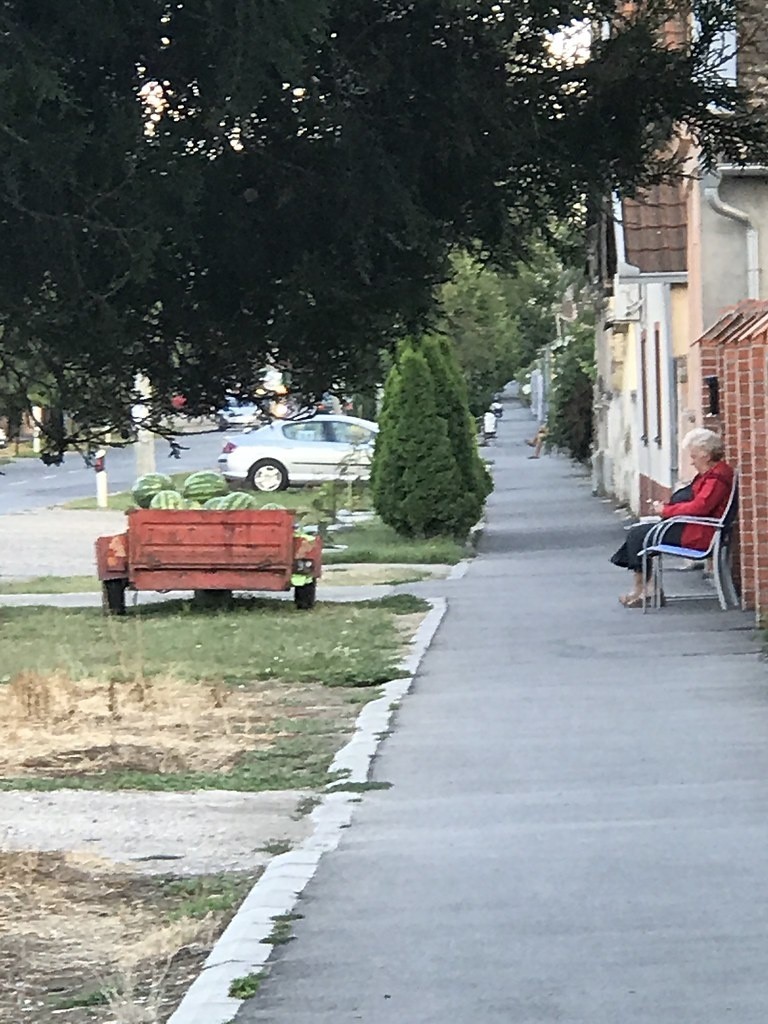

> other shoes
xmin=618 ymin=587 xmax=665 ymax=608
xmin=525 ymin=439 xmax=535 ymax=447
xmin=527 ymin=456 xmax=539 ymax=459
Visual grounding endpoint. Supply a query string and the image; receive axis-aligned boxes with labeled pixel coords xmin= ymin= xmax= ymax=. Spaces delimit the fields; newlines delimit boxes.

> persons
xmin=609 ymin=428 xmax=736 ymax=609
xmin=524 ymin=426 xmax=547 ymax=459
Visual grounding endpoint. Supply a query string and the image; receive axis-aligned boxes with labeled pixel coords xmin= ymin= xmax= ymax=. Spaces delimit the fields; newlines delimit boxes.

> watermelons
xmin=132 ymin=470 xmax=288 ymax=510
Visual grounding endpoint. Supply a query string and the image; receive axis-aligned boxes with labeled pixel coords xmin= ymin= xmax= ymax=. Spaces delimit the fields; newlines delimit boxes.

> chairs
xmin=623 ymin=467 xmax=740 ymax=611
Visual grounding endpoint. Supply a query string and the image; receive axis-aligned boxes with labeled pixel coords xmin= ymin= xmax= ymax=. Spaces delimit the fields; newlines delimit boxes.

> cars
xmin=216 ymin=397 xmax=264 ymax=432
xmin=218 ymin=415 xmax=380 ymax=494
